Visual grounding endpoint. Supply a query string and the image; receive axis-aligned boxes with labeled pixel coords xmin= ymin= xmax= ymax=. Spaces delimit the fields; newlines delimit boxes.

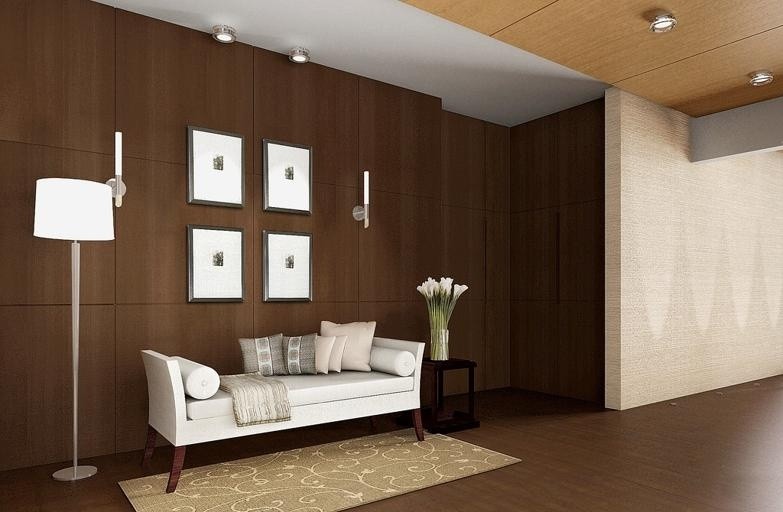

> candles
xmin=112 ymin=131 xmax=124 ymax=177
xmin=361 ymin=169 xmax=370 ymax=205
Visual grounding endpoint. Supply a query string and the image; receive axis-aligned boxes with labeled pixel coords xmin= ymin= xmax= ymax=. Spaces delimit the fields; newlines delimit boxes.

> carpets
xmin=115 ymin=424 xmax=523 ymax=512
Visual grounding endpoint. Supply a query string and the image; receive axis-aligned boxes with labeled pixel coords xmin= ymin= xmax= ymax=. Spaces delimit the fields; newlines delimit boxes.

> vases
xmin=427 ymin=328 xmax=450 ymax=362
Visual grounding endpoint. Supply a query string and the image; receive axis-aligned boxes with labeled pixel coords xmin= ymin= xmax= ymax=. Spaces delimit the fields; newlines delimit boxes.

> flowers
xmin=414 ymin=274 xmax=470 ymax=362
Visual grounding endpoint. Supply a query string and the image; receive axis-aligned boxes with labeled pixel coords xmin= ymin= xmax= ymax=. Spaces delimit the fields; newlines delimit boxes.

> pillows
xmin=168 ymin=353 xmax=221 ymax=402
xmin=318 ymin=317 xmax=376 ymax=374
xmin=278 ymin=332 xmax=319 ymax=377
xmin=313 ymin=335 xmax=336 ymax=375
xmin=369 ymin=344 xmax=416 ymax=377
xmin=236 ymin=330 xmax=287 ymax=379
xmin=324 ymin=334 xmax=348 ymax=375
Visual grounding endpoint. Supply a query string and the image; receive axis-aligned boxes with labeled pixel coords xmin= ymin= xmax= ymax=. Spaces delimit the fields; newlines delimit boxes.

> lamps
xmin=28 ymin=175 xmax=114 ymax=486
xmin=286 ymin=46 xmax=312 ymax=65
xmin=744 ymin=68 xmax=776 ymax=90
xmin=209 ymin=24 xmax=238 ymax=45
xmin=646 ymin=12 xmax=678 ymax=34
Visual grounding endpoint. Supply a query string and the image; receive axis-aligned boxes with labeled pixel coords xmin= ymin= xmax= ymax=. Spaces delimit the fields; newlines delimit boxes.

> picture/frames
xmin=182 ymin=222 xmax=245 ymax=306
xmin=257 ymin=227 xmax=314 ymax=304
xmin=183 ymin=121 xmax=246 ymax=212
xmin=257 ymin=135 xmax=314 ymax=219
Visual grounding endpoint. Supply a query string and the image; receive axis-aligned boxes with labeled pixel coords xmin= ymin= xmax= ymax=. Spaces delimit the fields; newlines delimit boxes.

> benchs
xmin=133 ymin=335 xmax=427 ymax=495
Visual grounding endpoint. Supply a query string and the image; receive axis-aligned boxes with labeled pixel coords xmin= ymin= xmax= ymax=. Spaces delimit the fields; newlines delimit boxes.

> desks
xmin=418 ymin=357 xmax=480 ymax=434
